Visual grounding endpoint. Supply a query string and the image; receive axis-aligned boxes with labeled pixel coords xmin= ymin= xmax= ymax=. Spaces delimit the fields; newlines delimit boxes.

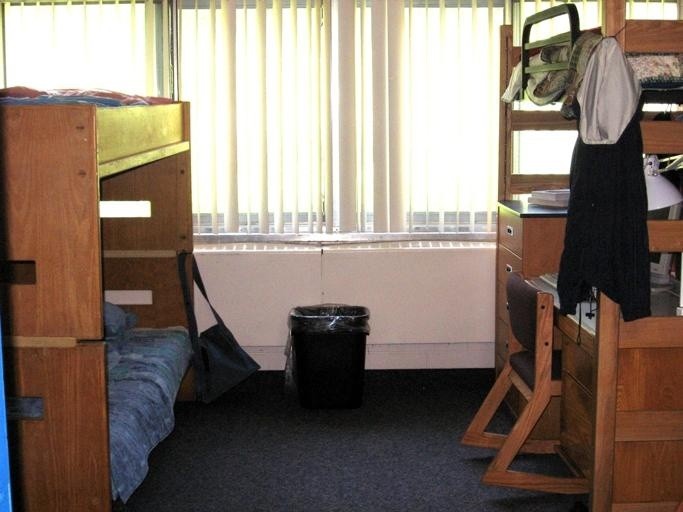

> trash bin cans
xmin=288 ymin=303 xmax=370 ymax=409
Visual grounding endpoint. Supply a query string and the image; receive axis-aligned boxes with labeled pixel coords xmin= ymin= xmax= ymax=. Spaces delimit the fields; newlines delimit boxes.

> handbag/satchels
xmin=193 ymin=321 xmax=261 ymax=405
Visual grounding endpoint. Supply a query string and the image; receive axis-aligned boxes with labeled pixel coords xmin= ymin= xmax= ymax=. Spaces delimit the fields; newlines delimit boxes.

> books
xmin=528 ymin=186 xmax=576 ymax=208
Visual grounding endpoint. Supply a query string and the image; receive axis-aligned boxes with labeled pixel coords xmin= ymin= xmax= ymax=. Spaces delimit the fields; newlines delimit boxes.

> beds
xmin=461 ymin=2 xmax=681 ymax=512
xmin=4 ymin=80 xmax=199 ymax=511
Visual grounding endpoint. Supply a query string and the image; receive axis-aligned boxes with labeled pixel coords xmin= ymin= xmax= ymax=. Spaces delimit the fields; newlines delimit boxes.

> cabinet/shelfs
xmin=485 ymin=194 xmax=566 ymax=437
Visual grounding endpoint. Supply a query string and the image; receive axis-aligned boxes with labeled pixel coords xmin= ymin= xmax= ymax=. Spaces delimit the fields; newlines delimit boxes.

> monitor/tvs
xmin=646 ymin=203 xmax=683 ymax=292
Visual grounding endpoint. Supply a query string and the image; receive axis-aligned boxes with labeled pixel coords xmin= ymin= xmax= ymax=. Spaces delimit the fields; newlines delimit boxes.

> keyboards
xmin=540 ymin=272 xmax=559 ymax=289
